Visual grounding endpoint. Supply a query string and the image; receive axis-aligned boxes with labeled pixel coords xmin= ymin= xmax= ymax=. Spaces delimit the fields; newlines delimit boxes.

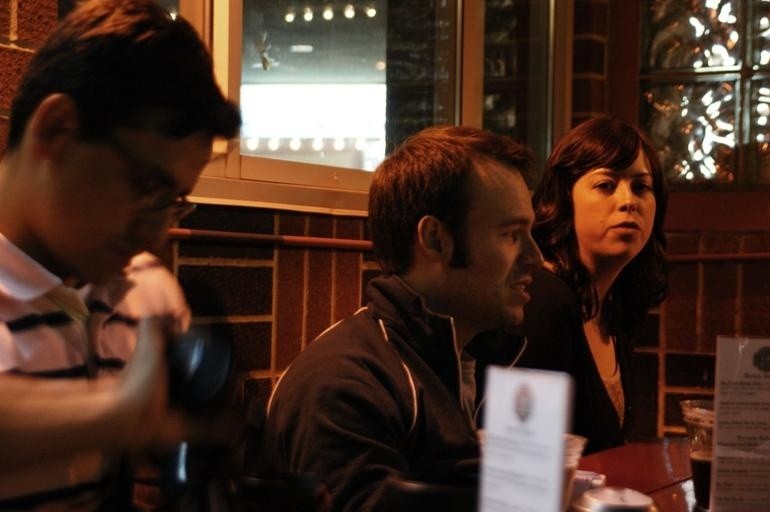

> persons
xmin=0 ymin=0 xmax=242 ymax=510
xmin=459 ymin=114 xmax=674 ymax=474
xmin=255 ymin=119 xmax=581 ymax=512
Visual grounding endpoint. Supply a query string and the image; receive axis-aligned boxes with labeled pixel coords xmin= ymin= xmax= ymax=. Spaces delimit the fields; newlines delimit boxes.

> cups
xmin=475 ymin=429 xmax=587 ymax=512
xmin=680 ymin=398 xmax=715 ymax=512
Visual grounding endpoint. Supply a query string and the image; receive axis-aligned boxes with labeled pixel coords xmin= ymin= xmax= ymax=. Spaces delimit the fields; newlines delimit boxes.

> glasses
xmin=103 ymin=132 xmax=196 ymax=222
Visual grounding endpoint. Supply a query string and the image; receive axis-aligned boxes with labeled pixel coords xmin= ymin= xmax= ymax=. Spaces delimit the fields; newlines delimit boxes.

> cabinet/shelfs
xmin=385 ymin=0 xmax=611 ymax=172
